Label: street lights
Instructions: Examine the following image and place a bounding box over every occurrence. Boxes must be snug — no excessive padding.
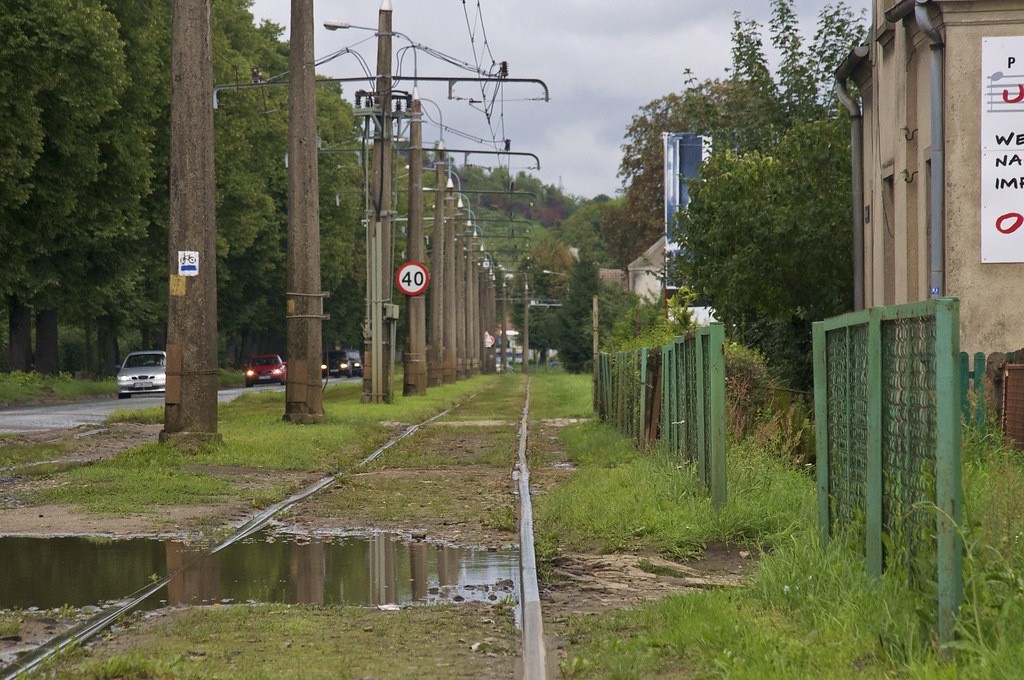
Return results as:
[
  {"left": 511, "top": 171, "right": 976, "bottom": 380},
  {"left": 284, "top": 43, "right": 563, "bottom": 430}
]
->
[
  {"left": 543, "top": 270, "right": 569, "bottom": 297},
  {"left": 322, "top": 17, "right": 392, "bottom": 403}
]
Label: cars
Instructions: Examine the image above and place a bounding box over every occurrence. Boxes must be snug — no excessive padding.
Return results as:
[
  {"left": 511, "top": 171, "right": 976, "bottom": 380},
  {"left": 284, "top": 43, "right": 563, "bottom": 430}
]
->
[
  {"left": 321, "top": 349, "right": 352, "bottom": 379},
  {"left": 244, "top": 354, "right": 287, "bottom": 388},
  {"left": 113, "top": 351, "right": 167, "bottom": 399},
  {"left": 344, "top": 348, "right": 364, "bottom": 377}
]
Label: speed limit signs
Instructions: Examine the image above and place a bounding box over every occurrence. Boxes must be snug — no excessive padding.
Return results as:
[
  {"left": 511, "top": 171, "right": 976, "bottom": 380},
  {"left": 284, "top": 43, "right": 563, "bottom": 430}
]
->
[{"left": 394, "top": 261, "right": 431, "bottom": 296}]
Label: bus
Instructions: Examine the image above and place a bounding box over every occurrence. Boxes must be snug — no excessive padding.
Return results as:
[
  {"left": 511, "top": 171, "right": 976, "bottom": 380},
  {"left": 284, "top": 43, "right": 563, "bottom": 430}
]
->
[{"left": 497, "top": 330, "right": 559, "bottom": 365}]
